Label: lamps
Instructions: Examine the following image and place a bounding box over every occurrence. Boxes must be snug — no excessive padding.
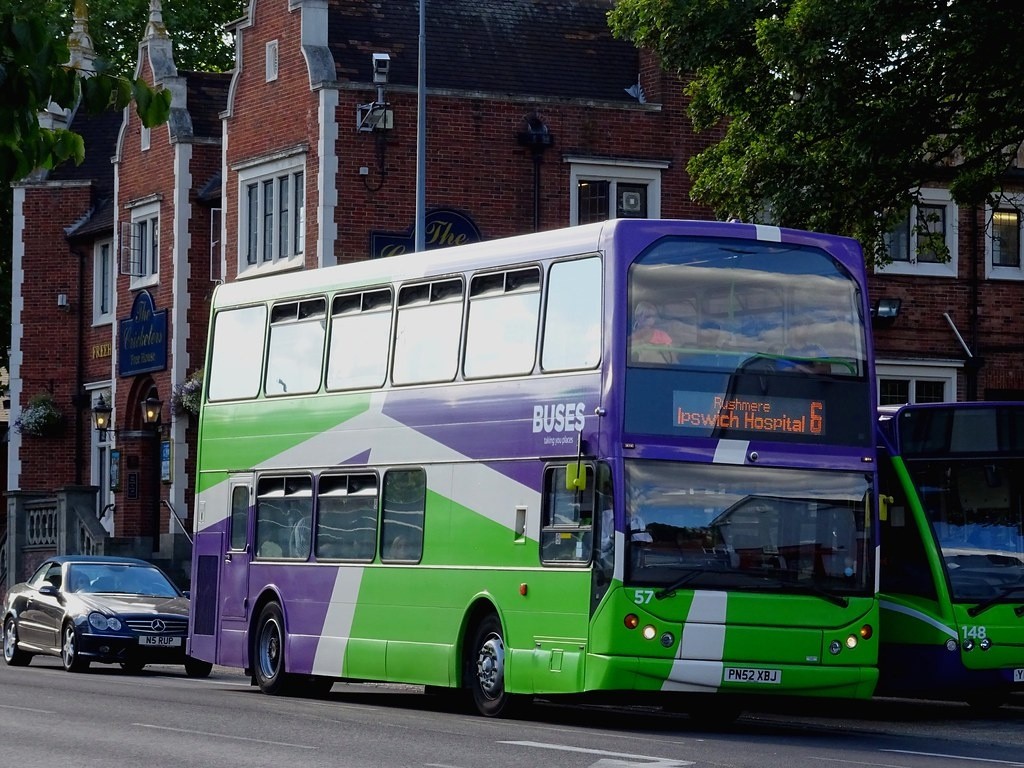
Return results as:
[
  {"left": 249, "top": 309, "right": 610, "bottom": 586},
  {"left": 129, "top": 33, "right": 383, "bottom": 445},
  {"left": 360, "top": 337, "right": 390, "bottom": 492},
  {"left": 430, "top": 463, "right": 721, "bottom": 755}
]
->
[
  {"left": 869, "top": 297, "right": 903, "bottom": 327},
  {"left": 89, "top": 393, "right": 118, "bottom": 436},
  {"left": 57, "top": 293, "right": 68, "bottom": 310},
  {"left": 139, "top": 389, "right": 172, "bottom": 428}
]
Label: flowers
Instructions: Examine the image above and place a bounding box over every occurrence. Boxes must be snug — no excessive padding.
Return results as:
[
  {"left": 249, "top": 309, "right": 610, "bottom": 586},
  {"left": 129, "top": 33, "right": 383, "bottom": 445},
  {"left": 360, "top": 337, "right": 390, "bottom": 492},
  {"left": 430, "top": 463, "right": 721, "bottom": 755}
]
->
[
  {"left": 169, "top": 365, "right": 205, "bottom": 416},
  {"left": 8, "top": 392, "right": 64, "bottom": 436}
]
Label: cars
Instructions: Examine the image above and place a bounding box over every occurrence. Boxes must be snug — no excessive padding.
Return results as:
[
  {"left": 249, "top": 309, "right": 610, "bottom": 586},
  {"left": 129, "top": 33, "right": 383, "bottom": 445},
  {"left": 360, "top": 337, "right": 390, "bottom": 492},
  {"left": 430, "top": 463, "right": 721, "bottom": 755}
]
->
[{"left": 2, "top": 554, "right": 212, "bottom": 678}]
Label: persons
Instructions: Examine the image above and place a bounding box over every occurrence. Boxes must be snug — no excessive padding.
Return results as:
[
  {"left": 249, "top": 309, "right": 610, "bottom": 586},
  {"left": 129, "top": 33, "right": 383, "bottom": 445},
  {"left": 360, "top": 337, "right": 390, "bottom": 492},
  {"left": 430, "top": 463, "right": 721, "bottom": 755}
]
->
[
  {"left": 630, "top": 301, "right": 831, "bottom": 372},
  {"left": 600, "top": 487, "right": 646, "bottom": 567}
]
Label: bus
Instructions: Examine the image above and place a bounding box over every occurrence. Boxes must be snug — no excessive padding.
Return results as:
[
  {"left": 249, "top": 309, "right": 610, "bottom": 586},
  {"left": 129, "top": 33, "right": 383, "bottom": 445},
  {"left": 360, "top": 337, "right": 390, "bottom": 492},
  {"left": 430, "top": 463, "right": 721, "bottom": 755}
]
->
[
  {"left": 876, "top": 401, "right": 1024, "bottom": 711},
  {"left": 186, "top": 218, "right": 884, "bottom": 721}
]
